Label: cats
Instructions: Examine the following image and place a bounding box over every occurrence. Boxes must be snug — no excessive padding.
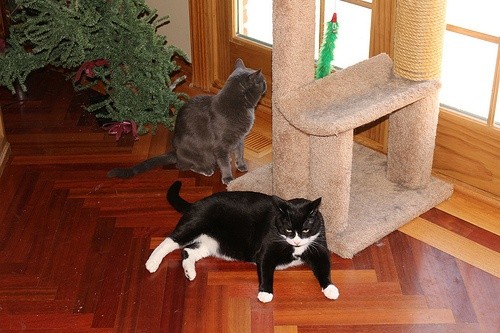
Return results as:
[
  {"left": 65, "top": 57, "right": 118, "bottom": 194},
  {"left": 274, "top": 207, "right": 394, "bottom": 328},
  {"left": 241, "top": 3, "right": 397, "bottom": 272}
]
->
[
  {"left": 144, "top": 179, "right": 340, "bottom": 304},
  {"left": 105, "top": 56, "right": 271, "bottom": 186}
]
